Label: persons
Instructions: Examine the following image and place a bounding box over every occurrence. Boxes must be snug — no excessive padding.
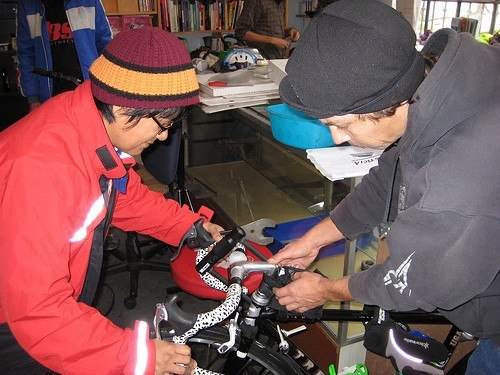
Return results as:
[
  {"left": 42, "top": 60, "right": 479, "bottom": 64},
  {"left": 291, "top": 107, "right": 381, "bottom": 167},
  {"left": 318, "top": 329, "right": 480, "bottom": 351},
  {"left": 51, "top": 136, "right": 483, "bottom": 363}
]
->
[
  {"left": 266, "top": 0, "right": 500, "bottom": 375},
  {"left": 235, "top": 0, "right": 301, "bottom": 59},
  {"left": 0, "top": 24, "right": 225, "bottom": 375},
  {"left": 16, "top": 0, "right": 114, "bottom": 113}
]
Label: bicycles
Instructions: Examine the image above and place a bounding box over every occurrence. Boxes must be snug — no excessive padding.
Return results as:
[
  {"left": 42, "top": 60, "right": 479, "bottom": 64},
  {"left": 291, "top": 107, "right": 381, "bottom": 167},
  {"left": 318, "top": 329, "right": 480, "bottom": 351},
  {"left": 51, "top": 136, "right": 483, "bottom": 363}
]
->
[{"left": 150, "top": 217, "right": 459, "bottom": 375}]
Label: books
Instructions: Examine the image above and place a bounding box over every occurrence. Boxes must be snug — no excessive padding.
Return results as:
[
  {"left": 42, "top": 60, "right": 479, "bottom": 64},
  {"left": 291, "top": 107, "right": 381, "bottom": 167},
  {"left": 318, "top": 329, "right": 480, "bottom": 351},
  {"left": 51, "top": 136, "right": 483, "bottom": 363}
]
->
[
  {"left": 451, "top": 17, "right": 478, "bottom": 36},
  {"left": 138, "top": 0, "right": 245, "bottom": 33}
]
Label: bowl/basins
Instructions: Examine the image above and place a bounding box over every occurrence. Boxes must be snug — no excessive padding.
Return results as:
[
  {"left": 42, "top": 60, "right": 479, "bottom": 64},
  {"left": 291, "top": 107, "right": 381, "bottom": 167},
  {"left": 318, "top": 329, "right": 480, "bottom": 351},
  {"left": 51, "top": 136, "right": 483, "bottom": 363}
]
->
[{"left": 264, "top": 104, "right": 336, "bottom": 149}]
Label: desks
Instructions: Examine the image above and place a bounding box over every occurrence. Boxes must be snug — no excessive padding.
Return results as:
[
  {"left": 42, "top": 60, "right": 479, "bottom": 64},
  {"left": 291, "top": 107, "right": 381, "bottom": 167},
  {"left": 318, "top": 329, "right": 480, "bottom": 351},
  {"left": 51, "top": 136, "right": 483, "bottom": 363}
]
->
[{"left": 232, "top": 107, "right": 409, "bottom": 347}]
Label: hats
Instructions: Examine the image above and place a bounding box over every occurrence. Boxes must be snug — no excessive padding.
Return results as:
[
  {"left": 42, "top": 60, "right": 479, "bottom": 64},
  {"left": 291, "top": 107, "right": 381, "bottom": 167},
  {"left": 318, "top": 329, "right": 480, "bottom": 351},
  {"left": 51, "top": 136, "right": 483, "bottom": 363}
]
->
[
  {"left": 279, "top": 0, "right": 425, "bottom": 118},
  {"left": 88, "top": 26, "right": 200, "bottom": 109}
]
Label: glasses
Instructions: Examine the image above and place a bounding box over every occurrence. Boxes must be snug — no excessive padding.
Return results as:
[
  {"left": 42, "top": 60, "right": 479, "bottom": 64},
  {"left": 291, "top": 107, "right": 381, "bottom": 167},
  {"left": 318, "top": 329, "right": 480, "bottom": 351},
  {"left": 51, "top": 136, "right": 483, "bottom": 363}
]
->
[{"left": 148, "top": 113, "right": 174, "bottom": 135}]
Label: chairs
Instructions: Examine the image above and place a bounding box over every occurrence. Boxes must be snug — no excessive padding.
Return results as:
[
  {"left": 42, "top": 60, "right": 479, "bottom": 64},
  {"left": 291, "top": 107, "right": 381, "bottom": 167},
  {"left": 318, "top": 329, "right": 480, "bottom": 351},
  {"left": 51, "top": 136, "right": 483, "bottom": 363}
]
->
[{"left": 95, "top": 112, "right": 186, "bottom": 308}]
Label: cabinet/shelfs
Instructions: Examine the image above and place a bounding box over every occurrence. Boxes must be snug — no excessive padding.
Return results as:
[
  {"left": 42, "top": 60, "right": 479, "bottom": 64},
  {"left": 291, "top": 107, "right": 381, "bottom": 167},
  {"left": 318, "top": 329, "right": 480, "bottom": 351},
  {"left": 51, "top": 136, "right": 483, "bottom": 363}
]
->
[{"left": 101, "top": 0, "right": 289, "bottom": 60}]
